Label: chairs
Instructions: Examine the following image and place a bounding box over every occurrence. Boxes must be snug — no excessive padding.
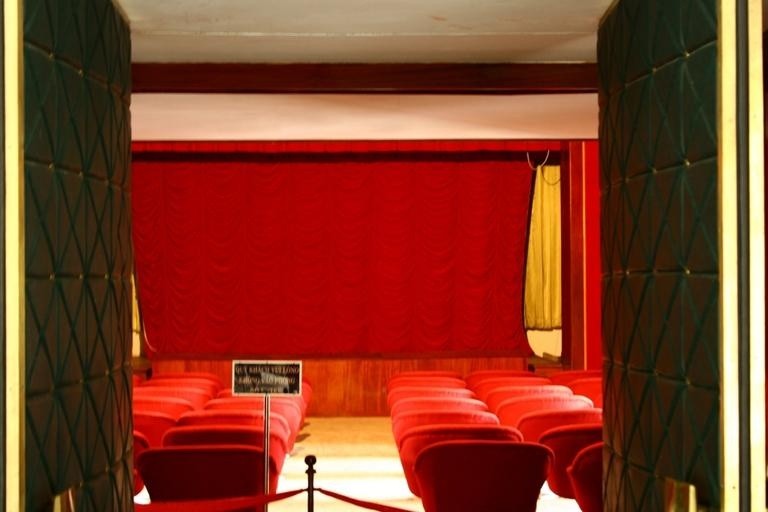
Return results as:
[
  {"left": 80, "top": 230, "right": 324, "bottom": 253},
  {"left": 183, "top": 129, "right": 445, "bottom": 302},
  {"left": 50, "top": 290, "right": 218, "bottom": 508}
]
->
[
  {"left": 126, "top": 365, "right": 316, "bottom": 509},
  {"left": 380, "top": 365, "right": 607, "bottom": 512}
]
